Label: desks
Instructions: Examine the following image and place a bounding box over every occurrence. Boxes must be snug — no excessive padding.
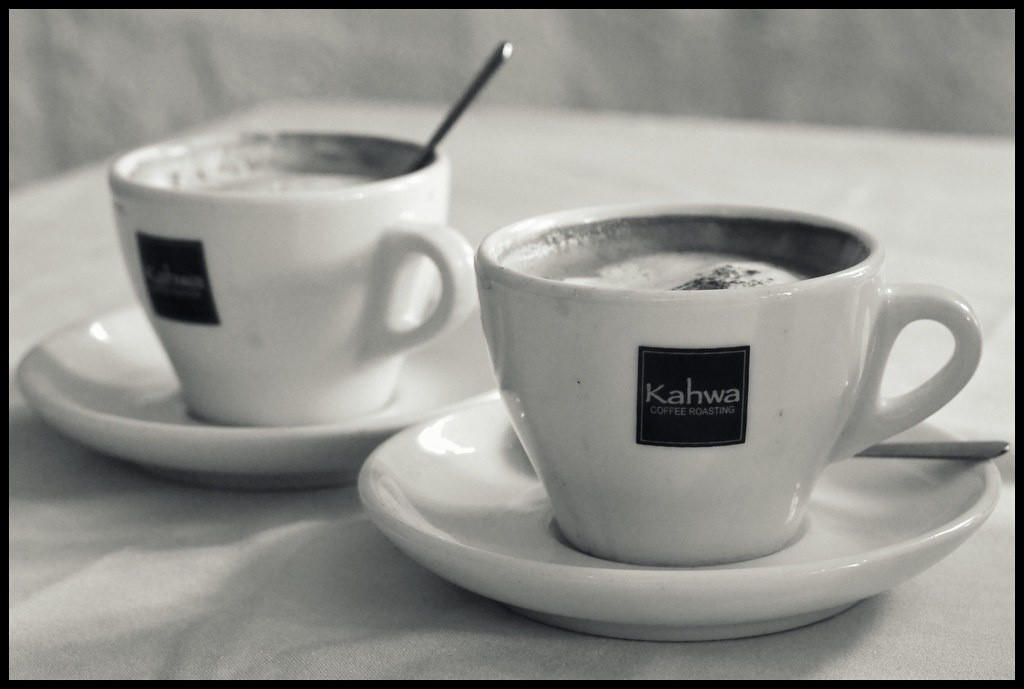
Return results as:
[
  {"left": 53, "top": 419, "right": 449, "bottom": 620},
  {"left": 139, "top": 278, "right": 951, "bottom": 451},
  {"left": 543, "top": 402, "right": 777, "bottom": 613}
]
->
[{"left": 9, "top": 99, "right": 1016, "bottom": 681}]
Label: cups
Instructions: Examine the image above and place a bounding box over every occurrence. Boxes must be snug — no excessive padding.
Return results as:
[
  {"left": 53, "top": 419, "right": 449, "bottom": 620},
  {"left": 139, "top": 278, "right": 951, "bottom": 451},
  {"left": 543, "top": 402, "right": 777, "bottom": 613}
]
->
[
  {"left": 476, "top": 206, "right": 983, "bottom": 565},
  {"left": 107, "top": 129, "right": 476, "bottom": 426}
]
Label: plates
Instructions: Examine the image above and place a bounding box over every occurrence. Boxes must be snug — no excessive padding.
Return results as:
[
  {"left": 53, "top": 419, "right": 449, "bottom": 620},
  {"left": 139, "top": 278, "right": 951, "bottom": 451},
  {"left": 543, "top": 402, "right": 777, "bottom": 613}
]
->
[
  {"left": 13, "top": 305, "right": 503, "bottom": 485},
  {"left": 358, "top": 394, "right": 1001, "bottom": 644}
]
